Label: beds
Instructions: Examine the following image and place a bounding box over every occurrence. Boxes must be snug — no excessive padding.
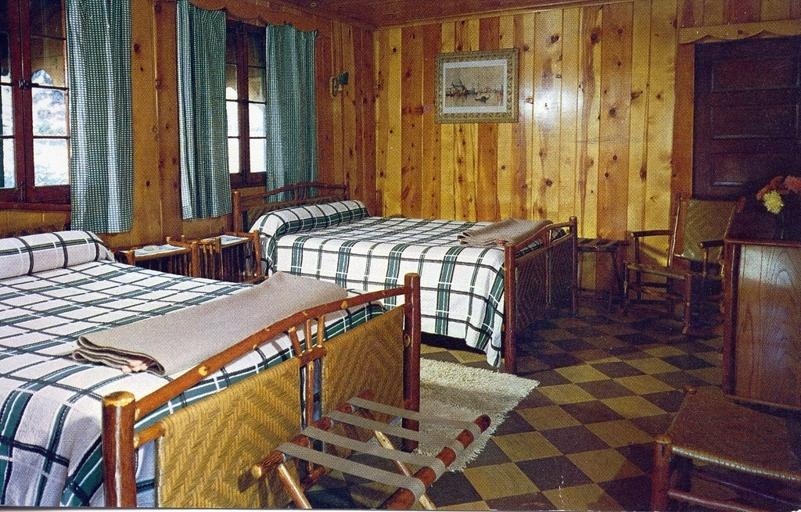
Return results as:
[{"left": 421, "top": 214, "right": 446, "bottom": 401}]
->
[
  {"left": 230, "top": 181, "right": 580, "bottom": 374},
  {"left": 0, "top": 208, "right": 421, "bottom": 507}
]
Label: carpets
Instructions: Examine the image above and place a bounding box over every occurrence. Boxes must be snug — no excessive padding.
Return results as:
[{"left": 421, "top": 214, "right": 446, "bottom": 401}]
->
[{"left": 365, "top": 351, "right": 540, "bottom": 473}]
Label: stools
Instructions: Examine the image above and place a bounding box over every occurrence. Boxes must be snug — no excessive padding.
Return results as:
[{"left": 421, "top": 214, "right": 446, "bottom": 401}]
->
[{"left": 248, "top": 389, "right": 490, "bottom": 511}]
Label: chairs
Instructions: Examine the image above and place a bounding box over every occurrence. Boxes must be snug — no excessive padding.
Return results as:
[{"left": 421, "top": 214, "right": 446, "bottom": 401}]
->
[{"left": 618, "top": 194, "right": 743, "bottom": 345}]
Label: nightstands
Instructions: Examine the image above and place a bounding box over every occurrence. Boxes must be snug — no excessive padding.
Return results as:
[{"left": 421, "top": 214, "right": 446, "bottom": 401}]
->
[{"left": 116, "top": 228, "right": 263, "bottom": 284}]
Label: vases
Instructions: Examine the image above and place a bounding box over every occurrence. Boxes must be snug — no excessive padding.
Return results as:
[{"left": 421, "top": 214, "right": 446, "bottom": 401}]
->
[{"left": 771, "top": 210, "right": 789, "bottom": 239}]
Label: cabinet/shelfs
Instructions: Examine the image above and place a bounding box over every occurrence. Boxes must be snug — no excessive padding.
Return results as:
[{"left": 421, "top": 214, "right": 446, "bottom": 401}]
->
[
  {"left": 691, "top": 35, "right": 801, "bottom": 202},
  {"left": 718, "top": 243, "right": 801, "bottom": 412}
]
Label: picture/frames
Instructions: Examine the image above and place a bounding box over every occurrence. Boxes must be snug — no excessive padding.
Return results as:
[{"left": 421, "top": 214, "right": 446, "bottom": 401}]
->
[{"left": 433, "top": 48, "right": 520, "bottom": 124}]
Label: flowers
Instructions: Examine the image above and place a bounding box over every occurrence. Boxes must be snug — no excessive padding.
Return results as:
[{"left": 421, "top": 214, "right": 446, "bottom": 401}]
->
[{"left": 753, "top": 174, "right": 801, "bottom": 216}]
